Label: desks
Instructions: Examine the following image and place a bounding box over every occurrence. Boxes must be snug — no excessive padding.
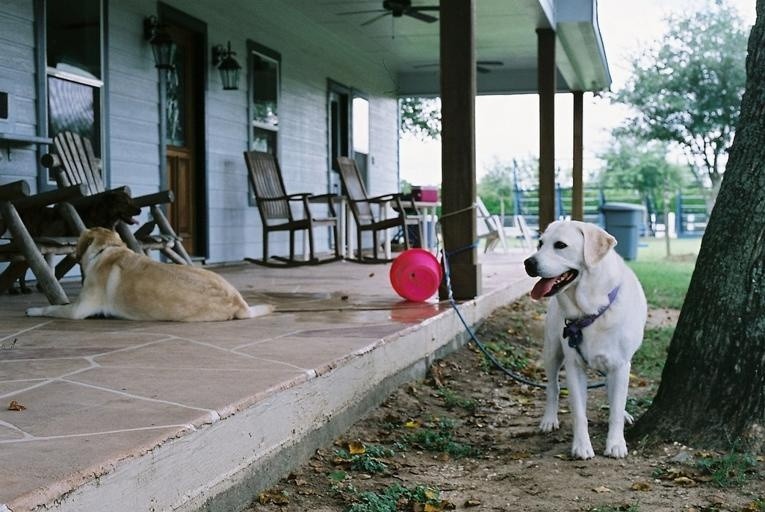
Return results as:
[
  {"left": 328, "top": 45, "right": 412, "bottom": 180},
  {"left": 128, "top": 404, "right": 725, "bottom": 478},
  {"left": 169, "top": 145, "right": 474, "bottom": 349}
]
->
[{"left": 390, "top": 200, "right": 442, "bottom": 253}]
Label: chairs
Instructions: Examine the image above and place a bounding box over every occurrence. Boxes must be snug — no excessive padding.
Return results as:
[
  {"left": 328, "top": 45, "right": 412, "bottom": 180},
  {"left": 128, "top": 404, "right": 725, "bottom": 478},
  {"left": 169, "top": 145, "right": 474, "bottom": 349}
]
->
[
  {"left": 243, "top": 150, "right": 341, "bottom": 268},
  {"left": 0, "top": 91, "right": 91, "bottom": 309},
  {"left": 337, "top": 156, "right": 422, "bottom": 265},
  {"left": 39, "top": 130, "right": 194, "bottom": 266}
]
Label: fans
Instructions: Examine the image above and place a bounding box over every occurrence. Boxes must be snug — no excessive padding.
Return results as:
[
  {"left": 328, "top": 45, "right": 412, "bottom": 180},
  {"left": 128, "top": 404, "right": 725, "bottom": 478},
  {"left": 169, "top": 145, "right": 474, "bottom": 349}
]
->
[
  {"left": 413, "top": 60, "right": 503, "bottom": 73},
  {"left": 331, "top": 0, "right": 440, "bottom": 27}
]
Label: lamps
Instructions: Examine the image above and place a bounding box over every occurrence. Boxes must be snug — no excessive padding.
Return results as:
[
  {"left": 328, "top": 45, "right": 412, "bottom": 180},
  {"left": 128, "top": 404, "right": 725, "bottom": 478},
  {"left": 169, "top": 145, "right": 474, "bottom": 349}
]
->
[
  {"left": 212, "top": 40, "right": 241, "bottom": 90},
  {"left": 144, "top": 14, "right": 181, "bottom": 70}
]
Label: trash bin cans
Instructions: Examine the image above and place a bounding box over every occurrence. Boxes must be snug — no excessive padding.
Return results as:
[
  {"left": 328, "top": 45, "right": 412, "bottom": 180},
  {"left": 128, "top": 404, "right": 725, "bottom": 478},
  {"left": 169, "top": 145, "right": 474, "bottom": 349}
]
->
[
  {"left": 596, "top": 202, "right": 646, "bottom": 260},
  {"left": 405, "top": 214, "right": 438, "bottom": 248}
]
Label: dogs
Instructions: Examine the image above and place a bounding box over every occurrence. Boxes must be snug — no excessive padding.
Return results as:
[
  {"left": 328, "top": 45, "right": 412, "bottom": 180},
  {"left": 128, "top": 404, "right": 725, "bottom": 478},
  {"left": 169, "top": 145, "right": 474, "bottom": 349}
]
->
[
  {"left": 25, "top": 227, "right": 279, "bottom": 323},
  {"left": 523, "top": 220, "right": 649, "bottom": 461}
]
